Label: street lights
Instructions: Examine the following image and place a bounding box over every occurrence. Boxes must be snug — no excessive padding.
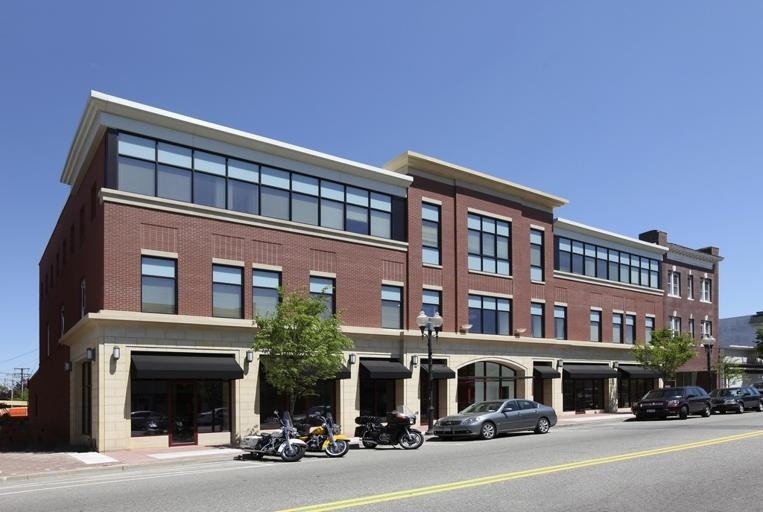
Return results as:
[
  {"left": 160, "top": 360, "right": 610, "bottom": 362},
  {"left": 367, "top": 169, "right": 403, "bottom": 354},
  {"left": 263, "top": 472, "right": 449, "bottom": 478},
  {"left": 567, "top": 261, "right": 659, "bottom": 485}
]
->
[
  {"left": 416, "top": 309, "right": 444, "bottom": 436},
  {"left": 702, "top": 335, "right": 716, "bottom": 392}
]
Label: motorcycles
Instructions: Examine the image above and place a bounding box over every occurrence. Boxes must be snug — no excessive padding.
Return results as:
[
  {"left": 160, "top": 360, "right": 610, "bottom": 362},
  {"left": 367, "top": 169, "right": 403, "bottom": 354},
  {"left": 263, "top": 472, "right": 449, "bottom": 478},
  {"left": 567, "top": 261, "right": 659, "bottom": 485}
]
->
[{"left": 234, "top": 405, "right": 424, "bottom": 462}]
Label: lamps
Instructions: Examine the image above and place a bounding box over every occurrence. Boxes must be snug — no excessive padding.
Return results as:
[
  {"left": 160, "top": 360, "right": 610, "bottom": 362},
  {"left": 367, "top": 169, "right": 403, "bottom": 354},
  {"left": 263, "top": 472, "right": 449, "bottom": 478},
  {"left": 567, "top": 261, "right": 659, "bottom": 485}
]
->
[
  {"left": 246, "top": 350, "right": 253, "bottom": 363},
  {"left": 411, "top": 355, "right": 417, "bottom": 365},
  {"left": 348, "top": 353, "right": 355, "bottom": 364},
  {"left": 112, "top": 346, "right": 120, "bottom": 360},
  {"left": 614, "top": 361, "right": 619, "bottom": 368},
  {"left": 558, "top": 359, "right": 563, "bottom": 367}
]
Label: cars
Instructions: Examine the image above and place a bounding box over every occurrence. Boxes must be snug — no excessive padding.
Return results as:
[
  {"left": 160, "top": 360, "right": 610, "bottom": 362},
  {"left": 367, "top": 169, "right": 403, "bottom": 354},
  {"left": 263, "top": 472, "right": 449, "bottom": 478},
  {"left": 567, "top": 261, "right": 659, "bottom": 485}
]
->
[
  {"left": 198, "top": 408, "right": 229, "bottom": 426},
  {"left": 433, "top": 399, "right": 557, "bottom": 440},
  {"left": 631, "top": 381, "right": 763, "bottom": 420},
  {"left": 131, "top": 410, "right": 167, "bottom": 431}
]
